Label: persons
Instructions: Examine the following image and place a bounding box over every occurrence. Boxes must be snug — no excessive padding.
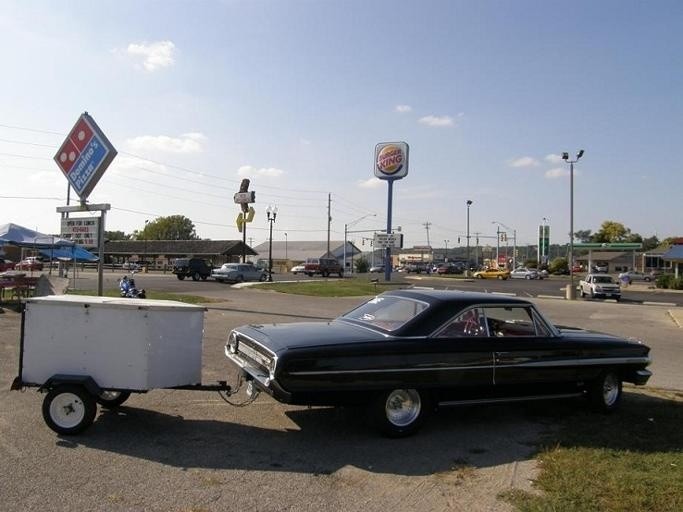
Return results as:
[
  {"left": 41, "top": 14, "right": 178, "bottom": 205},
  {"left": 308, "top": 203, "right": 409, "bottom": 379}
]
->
[
  {"left": 0, "top": 258, "right": 5, "bottom": 264},
  {"left": 433, "top": 265, "right": 437, "bottom": 273},
  {"left": 425, "top": 264, "right": 432, "bottom": 274},
  {"left": 119, "top": 275, "right": 128, "bottom": 297}
]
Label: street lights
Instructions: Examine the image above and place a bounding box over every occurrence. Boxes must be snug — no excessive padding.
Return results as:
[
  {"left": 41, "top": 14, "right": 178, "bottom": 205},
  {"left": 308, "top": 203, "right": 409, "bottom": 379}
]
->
[
  {"left": 562, "top": 149, "right": 584, "bottom": 284},
  {"left": 266, "top": 207, "right": 278, "bottom": 282},
  {"left": 466, "top": 200, "right": 472, "bottom": 270}
]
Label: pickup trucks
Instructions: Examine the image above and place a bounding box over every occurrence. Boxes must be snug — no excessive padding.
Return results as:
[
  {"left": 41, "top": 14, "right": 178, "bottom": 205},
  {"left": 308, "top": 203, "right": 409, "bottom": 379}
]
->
[{"left": 579, "top": 273, "right": 621, "bottom": 302}]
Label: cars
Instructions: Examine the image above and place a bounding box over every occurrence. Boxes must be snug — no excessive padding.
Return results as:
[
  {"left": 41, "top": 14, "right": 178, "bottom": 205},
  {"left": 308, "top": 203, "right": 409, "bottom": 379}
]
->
[
  {"left": 618, "top": 271, "right": 662, "bottom": 283},
  {"left": 594, "top": 263, "right": 608, "bottom": 273},
  {"left": 472, "top": 266, "right": 548, "bottom": 280},
  {"left": 290, "top": 258, "right": 344, "bottom": 278},
  {"left": 224, "top": 289, "right": 652, "bottom": 438},
  {"left": 392, "top": 262, "right": 464, "bottom": 274},
  {"left": 0, "top": 256, "right": 42, "bottom": 272},
  {"left": 370, "top": 264, "right": 386, "bottom": 273},
  {"left": 172, "top": 257, "right": 268, "bottom": 283}
]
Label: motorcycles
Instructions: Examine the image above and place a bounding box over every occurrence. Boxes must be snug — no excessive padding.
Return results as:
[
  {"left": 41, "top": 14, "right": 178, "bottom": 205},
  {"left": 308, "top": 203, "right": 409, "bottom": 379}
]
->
[{"left": 117, "top": 275, "right": 146, "bottom": 298}]
